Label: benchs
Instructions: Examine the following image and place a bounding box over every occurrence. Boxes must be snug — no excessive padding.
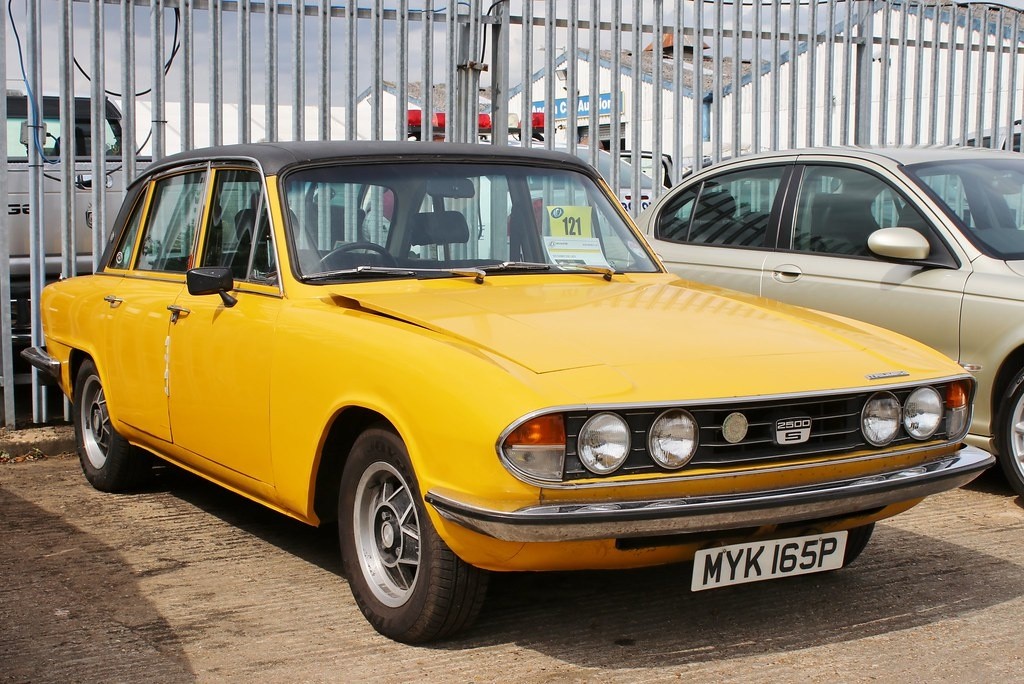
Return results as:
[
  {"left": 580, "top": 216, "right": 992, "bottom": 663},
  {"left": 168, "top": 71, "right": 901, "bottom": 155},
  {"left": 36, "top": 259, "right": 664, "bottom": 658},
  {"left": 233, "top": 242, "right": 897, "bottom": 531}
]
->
[
  {"left": 410, "top": 211, "right": 503, "bottom": 269},
  {"left": 694, "top": 192, "right": 770, "bottom": 246},
  {"left": 152, "top": 249, "right": 322, "bottom": 278}
]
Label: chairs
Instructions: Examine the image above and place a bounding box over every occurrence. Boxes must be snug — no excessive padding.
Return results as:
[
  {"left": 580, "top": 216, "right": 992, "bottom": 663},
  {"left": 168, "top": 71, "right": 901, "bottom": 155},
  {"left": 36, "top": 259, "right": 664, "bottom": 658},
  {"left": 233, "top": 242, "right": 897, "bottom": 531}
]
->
[
  {"left": 814, "top": 202, "right": 869, "bottom": 256},
  {"left": 897, "top": 203, "right": 929, "bottom": 233},
  {"left": 234, "top": 208, "right": 300, "bottom": 273},
  {"left": 55, "top": 127, "right": 86, "bottom": 156}
]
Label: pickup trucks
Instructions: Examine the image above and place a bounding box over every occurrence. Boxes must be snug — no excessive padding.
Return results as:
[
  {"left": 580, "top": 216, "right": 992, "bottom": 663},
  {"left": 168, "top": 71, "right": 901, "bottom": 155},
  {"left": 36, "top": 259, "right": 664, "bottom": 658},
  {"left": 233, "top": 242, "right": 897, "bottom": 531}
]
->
[{"left": 6, "top": 91, "right": 248, "bottom": 346}]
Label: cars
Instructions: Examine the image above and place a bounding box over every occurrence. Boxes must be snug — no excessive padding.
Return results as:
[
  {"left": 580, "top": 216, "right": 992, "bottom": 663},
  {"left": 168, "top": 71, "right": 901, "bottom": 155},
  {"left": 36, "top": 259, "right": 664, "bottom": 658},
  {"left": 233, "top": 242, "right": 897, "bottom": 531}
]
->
[
  {"left": 303, "top": 106, "right": 768, "bottom": 268},
  {"left": 829, "top": 118, "right": 1024, "bottom": 233},
  {"left": 602, "top": 146, "right": 1024, "bottom": 507},
  {"left": 15, "top": 140, "right": 999, "bottom": 647}
]
